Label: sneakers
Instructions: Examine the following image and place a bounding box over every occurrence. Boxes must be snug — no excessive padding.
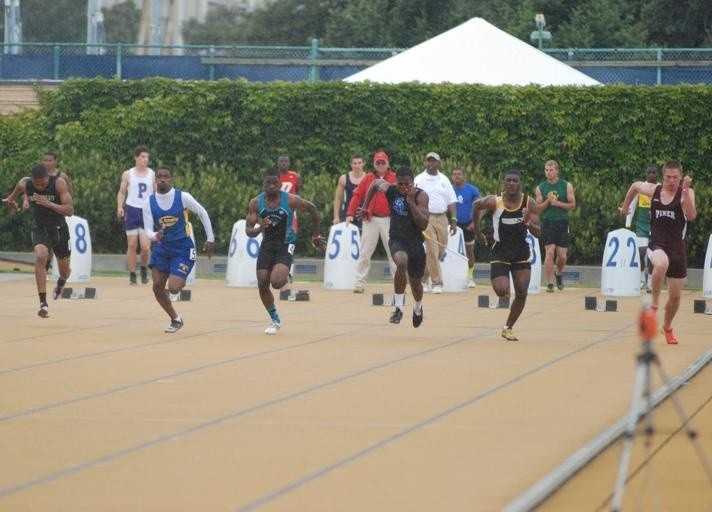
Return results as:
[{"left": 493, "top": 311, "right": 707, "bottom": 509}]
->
[
  {"left": 660, "top": 325, "right": 679, "bottom": 344},
  {"left": 38, "top": 277, "right": 184, "bottom": 332},
  {"left": 264, "top": 319, "right": 282, "bottom": 334},
  {"left": 353, "top": 270, "right": 563, "bottom": 341}
]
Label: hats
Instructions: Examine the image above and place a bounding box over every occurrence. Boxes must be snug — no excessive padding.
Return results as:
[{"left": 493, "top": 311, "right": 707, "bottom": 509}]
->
[
  {"left": 425, "top": 152, "right": 441, "bottom": 162},
  {"left": 373, "top": 152, "right": 389, "bottom": 161}
]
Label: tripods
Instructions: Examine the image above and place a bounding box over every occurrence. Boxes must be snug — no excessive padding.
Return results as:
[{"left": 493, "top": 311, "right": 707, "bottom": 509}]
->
[{"left": 610, "top": 300, "right": 712, "bottom": 512}]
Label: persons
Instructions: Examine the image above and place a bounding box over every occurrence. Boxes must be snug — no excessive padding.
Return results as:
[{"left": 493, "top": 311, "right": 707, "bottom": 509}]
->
[
  {"left": 620, "top": 159, "right": 698, "bottom": 344},
  {"left": 472, "top": 173, "right": 542, "bottom": 342},
  {"left": 625, "top": 166, "right": 661, "bottom": 294},
  {"left": 332, "top": 153, "right": 486, "bottom": 330},
  {"left": 22, "top": 151, "right": 72, "bottom": 281},
  {"left": 117, "top": 145, "right": 157, "bottom": 286},
  {"left": 143, "top": 166, "right": 215, "bottom": 333},
  {"left": 275, "top": 154, "right": 304, "bottom": 284},
  {"left": 245, "top": 167, "right": 327, "bottom": 334},
  {"left": 535, "top": 159, "right": 576, "bottom": 293},
  {"left": 2, "top": 163, "right": 75, "bottom": 318}
]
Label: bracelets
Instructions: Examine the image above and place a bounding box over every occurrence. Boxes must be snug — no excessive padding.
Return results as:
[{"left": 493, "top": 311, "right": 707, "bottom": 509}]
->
[
  {"left": 683, "top": 188, "right": 690, "bottom": 193},
  {"left": 556, "top": 201, "right": 560, "bottom": 208},
  {"left": 312, "top": 234, "right": 320, "bottom": 241},
  {"left": 525, "top": 219, "right": 532, "bottom": 226}
]
